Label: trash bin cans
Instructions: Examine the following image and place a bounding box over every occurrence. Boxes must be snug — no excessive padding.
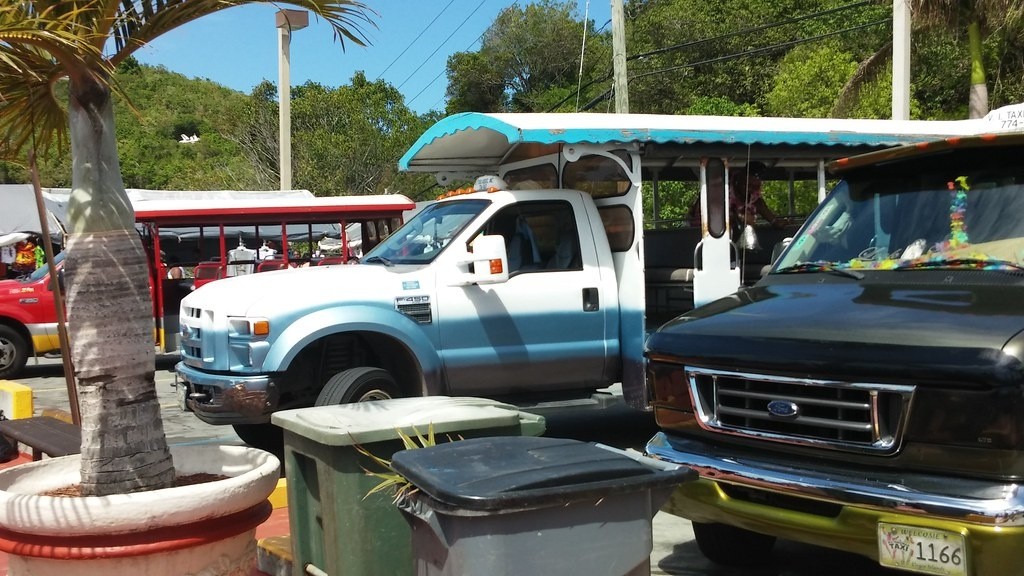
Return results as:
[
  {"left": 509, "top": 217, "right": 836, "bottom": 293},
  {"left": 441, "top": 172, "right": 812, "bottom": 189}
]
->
[
  {"left": 390, "top": 438, "right": 701, "bottom": 576},
  {"left": 271, "top": 395, "right": 547, "bottom": 576}
]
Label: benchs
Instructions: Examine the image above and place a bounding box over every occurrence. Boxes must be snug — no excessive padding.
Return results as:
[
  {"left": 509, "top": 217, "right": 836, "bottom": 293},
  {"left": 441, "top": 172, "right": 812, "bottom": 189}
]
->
[
  {"left": 163, "top": 257, "right": 343, "bottom": 316},
  {"left": 0, "top": 416, "right": 82, "bottom": 461},
  {"left": 645, "top": 269, "right": 695, "bottom": 311},
  {"left": 741, "top": 225, "right": 800, "bottom": 281}
]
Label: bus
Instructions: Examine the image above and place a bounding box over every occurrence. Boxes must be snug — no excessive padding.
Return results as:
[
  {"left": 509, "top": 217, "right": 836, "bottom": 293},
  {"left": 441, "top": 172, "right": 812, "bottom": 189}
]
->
[
  {"left": 0, "top": 195, "right": 414, "bottom": 381},
  {"left": 174, "top": 110, "right": 993, "bottom": 462}
]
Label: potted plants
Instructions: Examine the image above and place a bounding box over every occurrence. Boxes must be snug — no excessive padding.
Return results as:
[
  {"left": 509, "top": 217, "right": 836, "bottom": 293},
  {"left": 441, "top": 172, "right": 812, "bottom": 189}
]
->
[{"left": 1, "top": 0, "right": 380, "bottom": 575}]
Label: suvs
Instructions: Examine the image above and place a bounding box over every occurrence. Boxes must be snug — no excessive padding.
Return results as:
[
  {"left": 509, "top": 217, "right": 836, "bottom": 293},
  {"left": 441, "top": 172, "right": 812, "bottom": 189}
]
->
[{"left": 645, "top": 129, "right": 1024, "bottom": 576}]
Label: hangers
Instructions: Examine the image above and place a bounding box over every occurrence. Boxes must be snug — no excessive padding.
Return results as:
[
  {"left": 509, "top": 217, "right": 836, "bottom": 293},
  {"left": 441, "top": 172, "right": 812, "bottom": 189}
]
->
[
  {"left": 254, "top": 241, "right": 274, "bottom": 251},
  {"left": 234, "top": 242, "right": 248, "bottom": 252}
]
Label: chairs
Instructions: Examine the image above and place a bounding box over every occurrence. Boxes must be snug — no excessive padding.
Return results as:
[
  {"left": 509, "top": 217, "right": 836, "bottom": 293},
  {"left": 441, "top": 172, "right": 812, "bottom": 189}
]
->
[
  {"left": 507, "top": 233, "right": 529, "bottom": 272},
  {"left": 545, "top": 231, "right": 578, "bottom": 270}
]
